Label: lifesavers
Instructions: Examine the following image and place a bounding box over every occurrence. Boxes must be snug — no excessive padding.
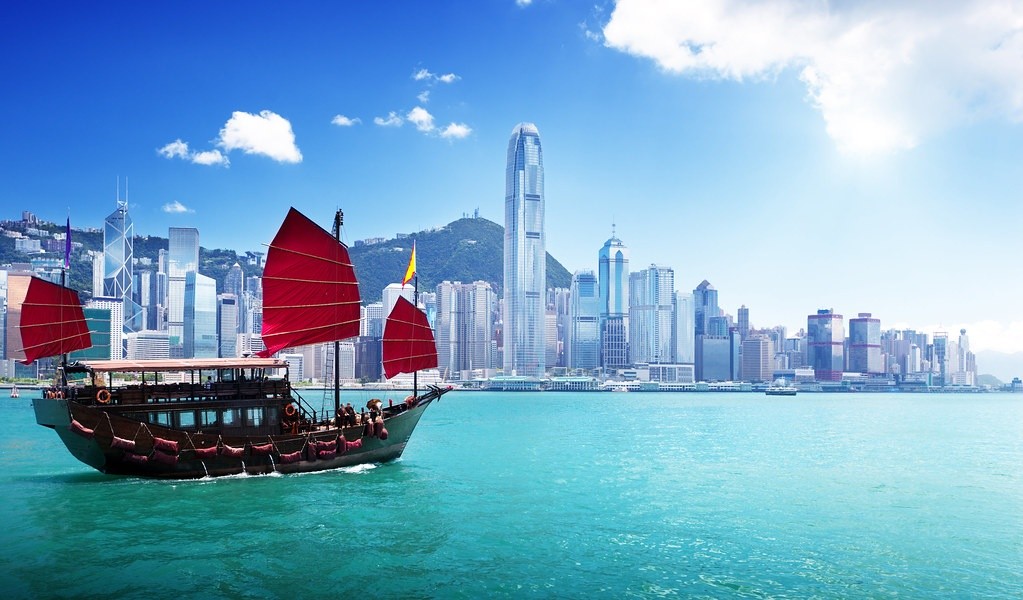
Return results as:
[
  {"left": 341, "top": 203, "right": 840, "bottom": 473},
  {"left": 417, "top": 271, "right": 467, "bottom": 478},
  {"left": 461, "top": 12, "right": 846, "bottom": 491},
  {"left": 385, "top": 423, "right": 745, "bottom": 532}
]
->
[
  {"left": 46, "top": 391, "right": 55, "bottom": 398},
  {"left": 97, "top": 390, "right": 110, "bottom": 403},
  {"left": 285, "top": 403, "right": 296, "bottom": 416},
  {"left": 57, "top": 391, "right": 65, "bottom": 400}
]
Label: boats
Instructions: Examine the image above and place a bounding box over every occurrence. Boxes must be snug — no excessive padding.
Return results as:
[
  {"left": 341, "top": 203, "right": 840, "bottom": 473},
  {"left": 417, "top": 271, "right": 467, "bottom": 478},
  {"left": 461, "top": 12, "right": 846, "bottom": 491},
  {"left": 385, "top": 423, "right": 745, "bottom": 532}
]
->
[
  {"left": 10, "top": 384, "right": 19, "bottom": 398},
  {"left": 8, "top": 207, "right": 453, "bottom": 479}
]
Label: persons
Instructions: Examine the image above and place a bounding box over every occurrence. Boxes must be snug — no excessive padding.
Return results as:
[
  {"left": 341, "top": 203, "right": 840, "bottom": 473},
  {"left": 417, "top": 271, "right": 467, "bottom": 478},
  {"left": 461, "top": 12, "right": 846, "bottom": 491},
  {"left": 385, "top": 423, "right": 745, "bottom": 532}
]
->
[
  {"left": 207, "top": 376, "right": 214, "bottom": 383},
  {"left": 345, "top": 403, "right": 354, "bottom": 428},
  {"left": 337, "top": 403, "right": 347, "bottom": 429},
  {"left": 241, "top": 371, "right": 246, "bottom": 380},
  {"left": 47, "top": 388, "right": 65, "bottom": 399}
]
